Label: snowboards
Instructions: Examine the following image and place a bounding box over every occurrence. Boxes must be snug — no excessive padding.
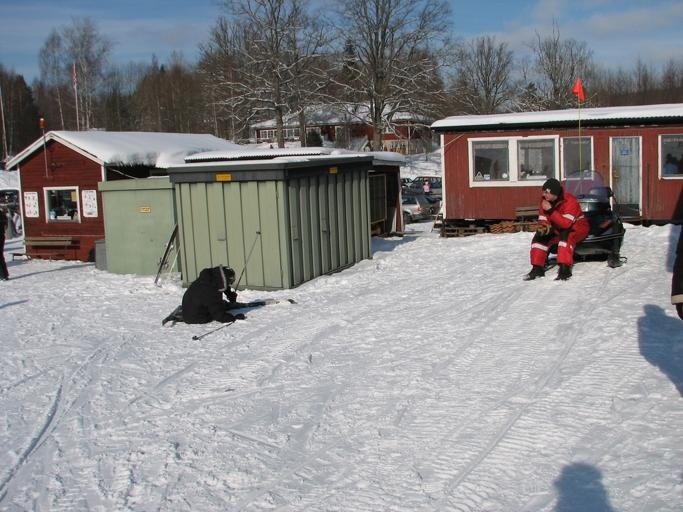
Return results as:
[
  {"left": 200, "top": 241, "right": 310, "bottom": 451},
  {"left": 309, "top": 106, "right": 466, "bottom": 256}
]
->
[{"left": 154, "top": 225, "right": 178, "bottom": 283}]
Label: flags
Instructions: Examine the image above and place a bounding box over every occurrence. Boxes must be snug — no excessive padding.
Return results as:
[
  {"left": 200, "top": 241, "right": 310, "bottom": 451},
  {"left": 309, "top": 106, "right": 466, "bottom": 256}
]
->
[{"left": 572, "top": 80, "right": 589, "bottom": 102}]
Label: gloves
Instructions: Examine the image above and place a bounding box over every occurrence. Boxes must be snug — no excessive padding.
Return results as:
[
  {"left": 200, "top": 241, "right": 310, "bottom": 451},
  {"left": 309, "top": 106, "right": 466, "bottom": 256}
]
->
[
  {"left": 536, "top": 223, "right": 552, "bottom": 238},
  {"left": 219, "top": 312, "right": 236, "bottom": 324},
  {"left": 226, "top": 291, "right": 237, "bottom": 302}
]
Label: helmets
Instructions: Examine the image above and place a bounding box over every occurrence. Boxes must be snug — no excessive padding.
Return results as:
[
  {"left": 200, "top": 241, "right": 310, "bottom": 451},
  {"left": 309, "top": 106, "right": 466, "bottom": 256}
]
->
[{"left": 222, "top": 265, "right": 236, "bottom": 279}]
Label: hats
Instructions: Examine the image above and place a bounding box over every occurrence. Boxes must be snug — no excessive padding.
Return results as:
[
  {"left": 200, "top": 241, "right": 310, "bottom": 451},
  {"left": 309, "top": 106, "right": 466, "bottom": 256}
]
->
[{"left": 541, "top": 177, "right": 561, "bottom": 195}]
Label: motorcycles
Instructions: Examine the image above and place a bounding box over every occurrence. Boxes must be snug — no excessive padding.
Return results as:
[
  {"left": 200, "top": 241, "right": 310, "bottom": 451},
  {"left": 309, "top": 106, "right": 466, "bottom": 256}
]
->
[{"left": 549, "top": 167, "right": 626, "bottom": 263}]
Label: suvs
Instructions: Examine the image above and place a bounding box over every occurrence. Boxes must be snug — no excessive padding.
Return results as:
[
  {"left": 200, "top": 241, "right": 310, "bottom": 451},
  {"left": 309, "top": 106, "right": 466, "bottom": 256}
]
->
[{"left": 402, "top": 176, "right": 441, "bottom": 203}]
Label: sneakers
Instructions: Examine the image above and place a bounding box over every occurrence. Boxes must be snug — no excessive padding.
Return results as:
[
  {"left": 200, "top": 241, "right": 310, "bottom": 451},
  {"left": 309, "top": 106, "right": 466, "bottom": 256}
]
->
[
  {"left": 553, "top": 265, "right": 574, "bottom": 282},
  {"left": 522, "top": 265, "right": 546, "bottom": 282}
]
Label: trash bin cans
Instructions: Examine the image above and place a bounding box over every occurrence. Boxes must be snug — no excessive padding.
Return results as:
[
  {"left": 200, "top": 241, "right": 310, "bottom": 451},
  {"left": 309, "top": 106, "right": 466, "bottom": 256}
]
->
[{"left": 94, "top": 240, "right": 106, "bottom": 270}]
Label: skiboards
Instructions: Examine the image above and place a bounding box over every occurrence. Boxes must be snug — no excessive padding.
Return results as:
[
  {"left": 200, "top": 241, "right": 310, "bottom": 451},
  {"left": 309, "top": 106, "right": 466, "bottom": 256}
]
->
[{"left": 162, "top": 299, "right": 298, "bottom": 326}]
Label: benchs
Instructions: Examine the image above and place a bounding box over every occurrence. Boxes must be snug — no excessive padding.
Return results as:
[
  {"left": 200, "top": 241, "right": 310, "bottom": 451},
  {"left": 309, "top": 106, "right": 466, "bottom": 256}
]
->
[
  {"left": 7, "top": 237, "right": 73, "bottom": 262},
  {"left": 515, "top": 203, "right": 540, "bottom": 232}
]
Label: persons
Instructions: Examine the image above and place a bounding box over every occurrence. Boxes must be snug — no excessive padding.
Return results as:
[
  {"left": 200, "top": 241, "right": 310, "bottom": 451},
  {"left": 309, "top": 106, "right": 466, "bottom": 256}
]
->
[
  {"left": 523, "top": 182, "right": 589, "bottom": 281},
  {"left": 422, "top": 181, "right": 430, "bottom": 194},
  {"left": 671, "top": 227, "right": 683, "bottom": 322},
  {"left": 0, "top": 211, "right": 13, "bottom": 282},
  {"left": 182, "top": 265, "right": 256, "bottom": 324}
]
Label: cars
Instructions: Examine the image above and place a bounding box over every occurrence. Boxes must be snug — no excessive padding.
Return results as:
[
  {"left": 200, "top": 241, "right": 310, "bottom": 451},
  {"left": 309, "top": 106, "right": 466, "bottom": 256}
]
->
[
  {"left": 0, "top": 189, "right": 19, "bottom": 211},
  {"left": 400, "top": 178, "right": 412, "bottom": 185}
]
